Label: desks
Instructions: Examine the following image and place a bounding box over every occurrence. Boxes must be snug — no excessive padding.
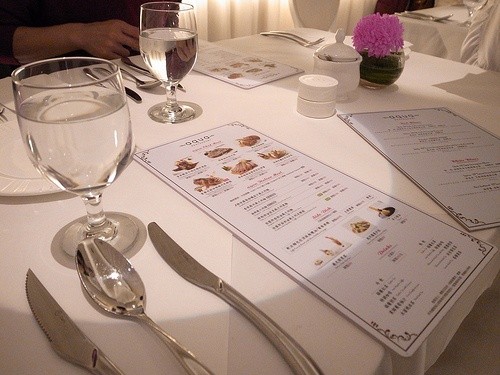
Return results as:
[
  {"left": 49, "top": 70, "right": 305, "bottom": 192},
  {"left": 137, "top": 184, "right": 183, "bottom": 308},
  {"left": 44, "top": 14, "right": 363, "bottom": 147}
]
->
[
  {"left": 391, "top": 6, "right": 477, "bottom": 63},
  {"left": 0, "top": 27, "right": 500, "bottom": 375}
]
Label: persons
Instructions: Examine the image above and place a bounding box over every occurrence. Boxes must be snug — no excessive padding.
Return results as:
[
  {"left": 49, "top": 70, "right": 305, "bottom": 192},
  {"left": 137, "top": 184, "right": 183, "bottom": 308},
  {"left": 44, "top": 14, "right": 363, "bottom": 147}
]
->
[{"left": 0, "top": 0, "right": 197, "bottom": 82}]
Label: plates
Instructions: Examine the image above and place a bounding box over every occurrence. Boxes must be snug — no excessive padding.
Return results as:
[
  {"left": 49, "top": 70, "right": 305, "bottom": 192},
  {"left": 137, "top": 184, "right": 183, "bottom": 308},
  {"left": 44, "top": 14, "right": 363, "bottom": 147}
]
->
[{"left": 0, "top": 74, "right": 69, "bottom": 196}]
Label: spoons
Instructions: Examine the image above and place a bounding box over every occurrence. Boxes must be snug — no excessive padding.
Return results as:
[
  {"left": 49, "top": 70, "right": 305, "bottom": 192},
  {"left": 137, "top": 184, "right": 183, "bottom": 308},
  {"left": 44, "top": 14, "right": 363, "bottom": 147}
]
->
[
  {"left": 75, "top": 239, "right": 215, "bottom": 375},
  {"left": 401, "top": 12, "right": 452, "bottom": 21},
  {"left": 120, "top": 67, "right": 162, "bottom": 89}
]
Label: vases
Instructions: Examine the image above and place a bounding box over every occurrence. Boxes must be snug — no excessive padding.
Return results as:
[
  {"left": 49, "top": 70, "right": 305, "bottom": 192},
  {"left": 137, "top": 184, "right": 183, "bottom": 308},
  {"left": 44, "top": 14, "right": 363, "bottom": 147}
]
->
[{"left": 358, "top": 47, "right": 405, "bottom": 87}]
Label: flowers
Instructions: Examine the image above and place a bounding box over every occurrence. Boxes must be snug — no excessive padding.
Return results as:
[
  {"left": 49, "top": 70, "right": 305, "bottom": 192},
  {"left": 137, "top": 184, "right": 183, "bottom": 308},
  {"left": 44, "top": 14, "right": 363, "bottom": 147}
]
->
[{"left": 351, "top": 12, "right": 405, "bottom": 58}]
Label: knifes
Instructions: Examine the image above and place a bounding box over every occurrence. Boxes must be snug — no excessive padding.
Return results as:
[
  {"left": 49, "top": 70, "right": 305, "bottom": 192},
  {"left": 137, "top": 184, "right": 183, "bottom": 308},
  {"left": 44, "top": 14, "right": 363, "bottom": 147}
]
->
[
  {"left": 121, "top": 55, "right": 183, "bottom": 89},
  {"left": 25, "top": 268, "right": 126, "bottom": 375},
  {"left": 148, "top": 221, "right": 324, "bottom": 375},
  {"left": 92, "top": 67, "right": 142, "bottom": 102}
]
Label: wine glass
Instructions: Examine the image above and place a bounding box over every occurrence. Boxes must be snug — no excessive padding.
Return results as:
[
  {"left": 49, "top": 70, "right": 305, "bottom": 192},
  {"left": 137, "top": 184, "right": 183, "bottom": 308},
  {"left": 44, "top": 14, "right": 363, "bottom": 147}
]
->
[
  {"left": 139, "top": 2, "right": 198, "bottom": 124},
  {"left": 11, "top": 56, "right": 138, "bottom": 255},
  {"left": 462, "top": 0, "right": 487, "bottom": 26}
]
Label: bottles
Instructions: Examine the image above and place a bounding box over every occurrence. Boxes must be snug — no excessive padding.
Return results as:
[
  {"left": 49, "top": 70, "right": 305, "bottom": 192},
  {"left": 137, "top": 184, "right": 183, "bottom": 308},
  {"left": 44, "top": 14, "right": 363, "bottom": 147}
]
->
[{"left": 312, "top": 29, "right": 362, "bottom": 102}]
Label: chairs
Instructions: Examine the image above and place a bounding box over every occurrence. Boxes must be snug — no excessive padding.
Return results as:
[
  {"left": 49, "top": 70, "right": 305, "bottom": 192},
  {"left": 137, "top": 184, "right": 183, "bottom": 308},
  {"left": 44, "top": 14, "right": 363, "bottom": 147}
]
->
[{"left": 287, "top": 0, "right": 340, "bottom": 31}]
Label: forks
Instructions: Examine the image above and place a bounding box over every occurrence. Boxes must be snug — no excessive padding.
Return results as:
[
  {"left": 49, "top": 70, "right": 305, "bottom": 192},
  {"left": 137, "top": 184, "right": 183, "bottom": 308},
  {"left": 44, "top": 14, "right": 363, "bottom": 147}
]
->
[{"left": 260, "top": 30, "right": 325, "bottom": 47}]
